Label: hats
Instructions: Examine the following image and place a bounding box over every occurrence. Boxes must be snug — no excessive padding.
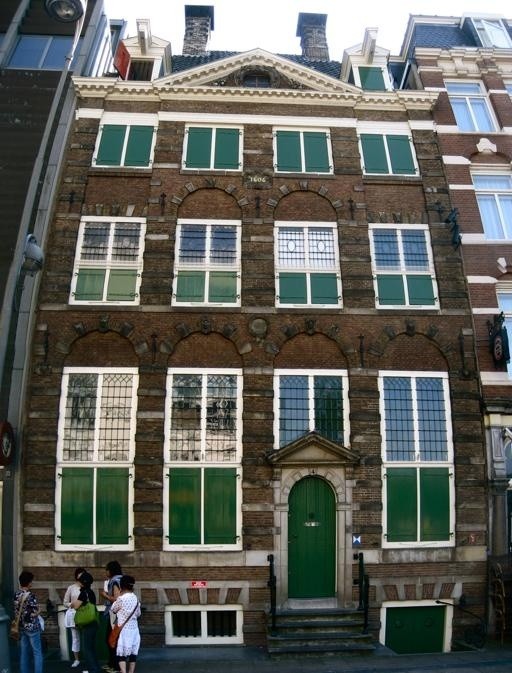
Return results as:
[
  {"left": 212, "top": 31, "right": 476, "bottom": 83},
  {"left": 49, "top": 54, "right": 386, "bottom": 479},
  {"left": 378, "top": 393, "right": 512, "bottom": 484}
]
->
[{"left": 77, "top": 573, "right": 93, "bottom": 585}]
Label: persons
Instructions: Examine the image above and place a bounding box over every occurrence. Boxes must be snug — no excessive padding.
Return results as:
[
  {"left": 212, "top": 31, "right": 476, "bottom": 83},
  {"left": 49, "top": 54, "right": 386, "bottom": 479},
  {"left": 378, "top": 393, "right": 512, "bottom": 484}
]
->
[
  {"left": 70, "top": 572, "right": 103, "bottom": 673},
  {"left": 62, "top": 568, "right": 87, "bottom": 669},
  {"left": 100, "top": 560, "right": 124, "bottom": 673},
  {"left": 110, "top": 574, "right": 142, "bottom": 673},
  {"left": 12, "top": 571, "right": 44, "bottom": 673}
]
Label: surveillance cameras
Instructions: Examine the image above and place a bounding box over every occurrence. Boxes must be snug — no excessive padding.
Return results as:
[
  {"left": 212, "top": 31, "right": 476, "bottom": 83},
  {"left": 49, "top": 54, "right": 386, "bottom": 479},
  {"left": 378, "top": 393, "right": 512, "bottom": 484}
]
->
[
  {"left": 444, "top": 206, "right": 460, "bottom": 223},
  {"left": 20, "top": 242, "right": 44, "bottom": 273}
]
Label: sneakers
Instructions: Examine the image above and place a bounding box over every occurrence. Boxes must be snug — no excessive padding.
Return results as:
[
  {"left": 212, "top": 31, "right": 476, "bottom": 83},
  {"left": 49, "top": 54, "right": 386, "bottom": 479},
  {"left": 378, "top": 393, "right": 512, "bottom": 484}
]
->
[
  {"left": 72, "top": 659, "right": 80, "bottom": 667},
  {"left": 102, "top": 665, "right": 119, "bottom": 673}
]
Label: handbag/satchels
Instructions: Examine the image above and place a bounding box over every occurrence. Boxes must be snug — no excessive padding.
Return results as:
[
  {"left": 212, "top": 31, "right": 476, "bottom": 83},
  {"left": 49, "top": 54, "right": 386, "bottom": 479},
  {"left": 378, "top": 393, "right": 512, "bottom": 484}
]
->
[
  {"left": 65, "top": 606, "right": 76, "bottom": 628},
  {"left": 9, "top": 620, "right": 21, "bottom": 641},
  {"left": 108, "top": 623, "right": 122, "bottom": 648},
  {"left": 74, "top": 602, "right": 96, "bottom": 625}
]
